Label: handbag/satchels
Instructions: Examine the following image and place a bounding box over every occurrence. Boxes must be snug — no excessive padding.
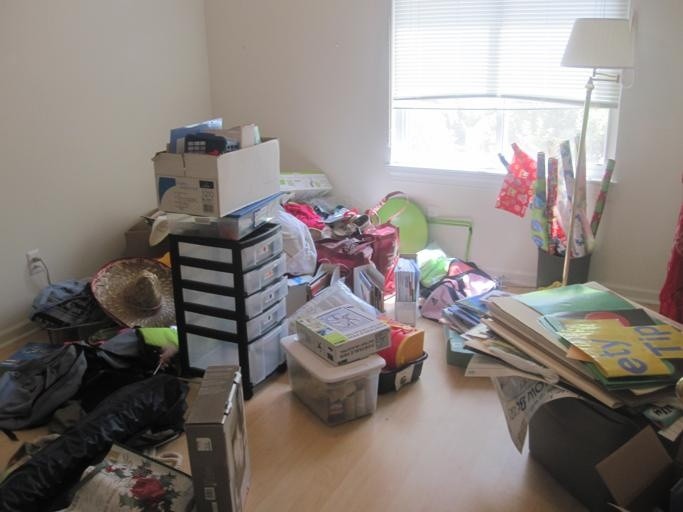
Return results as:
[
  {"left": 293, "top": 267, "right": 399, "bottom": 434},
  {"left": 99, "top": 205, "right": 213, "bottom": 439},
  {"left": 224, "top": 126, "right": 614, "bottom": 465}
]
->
[
  {"left": 0, "top": 372, "right": 184, "bottom": 512},
  {"left": 315, "top": 214, "right": 402, "bottom": 303},
  {"left": 417, "top": 256, "right": 501, "bottom": 325}
]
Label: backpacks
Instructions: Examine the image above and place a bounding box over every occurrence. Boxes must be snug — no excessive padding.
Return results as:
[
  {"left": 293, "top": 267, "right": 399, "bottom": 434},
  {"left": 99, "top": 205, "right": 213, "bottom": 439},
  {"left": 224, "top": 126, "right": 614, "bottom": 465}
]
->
[
  {"left": 28, "top": 278, "right": 114, "bottom": 335},
  {"left": 0, "top": 339, "right": 89, "bottom": 431}
]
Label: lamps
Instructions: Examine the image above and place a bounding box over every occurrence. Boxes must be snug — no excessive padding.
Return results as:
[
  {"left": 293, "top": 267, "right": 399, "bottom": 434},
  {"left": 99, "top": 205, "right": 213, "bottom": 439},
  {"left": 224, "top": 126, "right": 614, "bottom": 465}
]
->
[{"left": 561, "top": 17, "right": 635, "bottom": 293}]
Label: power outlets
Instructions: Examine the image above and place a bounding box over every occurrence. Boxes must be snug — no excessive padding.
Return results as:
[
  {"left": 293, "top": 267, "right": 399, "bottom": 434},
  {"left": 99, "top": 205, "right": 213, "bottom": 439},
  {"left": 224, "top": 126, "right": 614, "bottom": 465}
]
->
[{"left": 25, "top": 248, "right": 44, "bottom": 278}]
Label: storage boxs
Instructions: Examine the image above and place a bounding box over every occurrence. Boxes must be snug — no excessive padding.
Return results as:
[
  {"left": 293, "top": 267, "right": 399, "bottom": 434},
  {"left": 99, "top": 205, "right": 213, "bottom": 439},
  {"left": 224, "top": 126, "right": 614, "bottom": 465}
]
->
[
  {"left": 153, "top": 136, "right": 282, "bottom": 219},
  {"left": 295, "top": 303, "right": 394, "bottom": 367},
  {"left": 166, "top": 222, "right": 291, "bottom": 402},
  {"left": 158, "top": 193, "right": 283, "bottom": 242},
  {"left": 184, "top": 365, "right": 252, "bottom": 512},
  {"left": 520, "top": 376, "right": 682, "bottom": 511},
  {"left": 282, "top": 173, "right": 334, "bottom": 202},
  {"left": 288, "top": 271, "right": 330, "bottom": 316},
  {"left": 278, "top": 330, "right": 387, "bottom": 428}
]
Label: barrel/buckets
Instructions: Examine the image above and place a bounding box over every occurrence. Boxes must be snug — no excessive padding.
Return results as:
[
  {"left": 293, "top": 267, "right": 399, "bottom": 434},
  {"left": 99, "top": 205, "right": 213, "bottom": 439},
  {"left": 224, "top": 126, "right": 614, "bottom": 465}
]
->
[{"left": 537, "top": 247, "right": 590, "bottom": 287}]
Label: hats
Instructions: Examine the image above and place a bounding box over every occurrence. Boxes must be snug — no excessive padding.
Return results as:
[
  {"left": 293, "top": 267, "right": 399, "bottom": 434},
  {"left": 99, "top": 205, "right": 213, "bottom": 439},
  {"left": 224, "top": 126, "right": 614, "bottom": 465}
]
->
[{"left": 89, "top": 255, "right": 178, "bottom": 330}]
